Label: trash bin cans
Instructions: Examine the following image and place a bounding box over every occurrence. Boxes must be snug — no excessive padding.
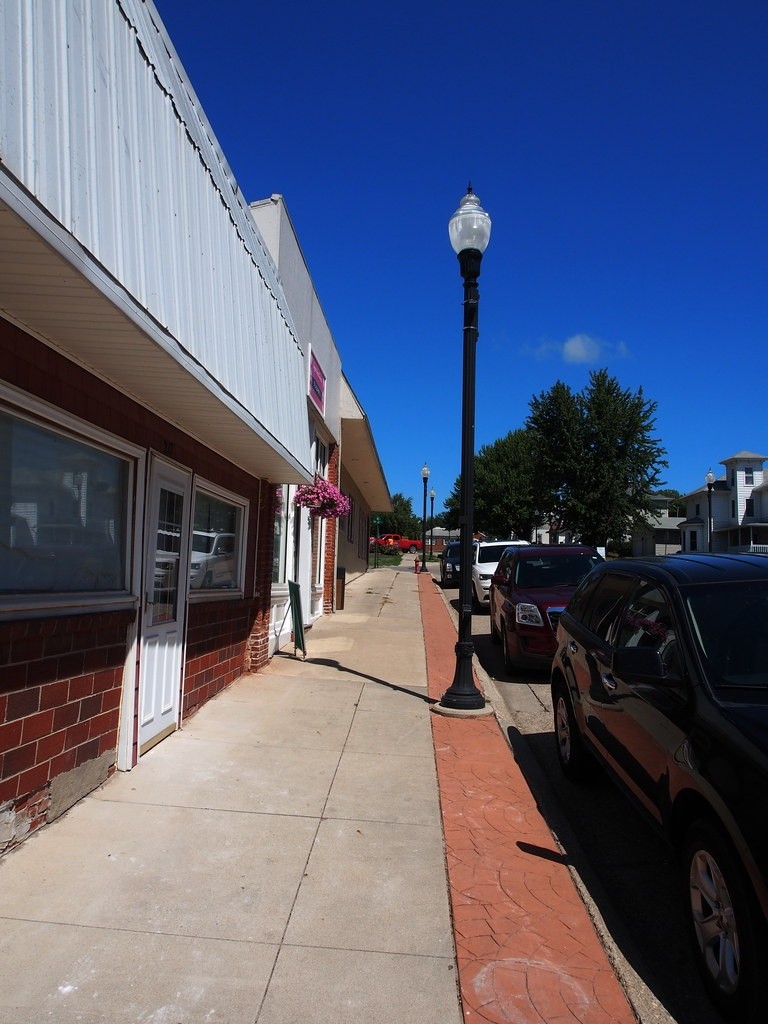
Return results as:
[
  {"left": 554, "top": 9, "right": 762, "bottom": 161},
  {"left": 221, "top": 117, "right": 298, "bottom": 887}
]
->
[{"left": 336, "top": 566, "right": 346, "bottom": 610}]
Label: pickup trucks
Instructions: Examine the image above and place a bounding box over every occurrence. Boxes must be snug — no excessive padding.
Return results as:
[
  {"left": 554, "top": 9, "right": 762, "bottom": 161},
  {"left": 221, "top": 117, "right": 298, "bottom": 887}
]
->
[{"left": 371, "top": 534, "right": 423, "bottom": 554}]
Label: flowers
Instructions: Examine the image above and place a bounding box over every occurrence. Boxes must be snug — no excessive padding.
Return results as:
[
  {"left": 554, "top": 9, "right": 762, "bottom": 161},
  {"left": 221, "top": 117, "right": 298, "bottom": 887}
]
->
[
  {"left": 292, "top": 474, "right": 350, "bottom": 520},
  {"left": 624, "top": 612, "right": 668, "bottom": 641}
]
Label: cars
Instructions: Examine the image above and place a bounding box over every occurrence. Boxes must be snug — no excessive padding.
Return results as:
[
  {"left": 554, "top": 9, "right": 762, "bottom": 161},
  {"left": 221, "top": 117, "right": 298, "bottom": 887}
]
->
[
  {"left": 437, "top": 542, "right": 463, "bottom": 587},
  {"left": 549, "top": 554, "right": 767, "bottom": 1016},
  {"left": 472, "top": 539, "right": 530, "bottom": 612}
]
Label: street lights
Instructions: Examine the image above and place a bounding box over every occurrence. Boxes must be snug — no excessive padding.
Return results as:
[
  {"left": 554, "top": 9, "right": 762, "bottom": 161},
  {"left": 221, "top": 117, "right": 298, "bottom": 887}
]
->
[
  {"left": 441, "top": 182, "right": 493, "bottom": 710},
  {"left": 420, "top": 462, "right": 430, "bottom": 572},
  {"left": 428, "top": 486, "right": 436, "bottom": 561}
]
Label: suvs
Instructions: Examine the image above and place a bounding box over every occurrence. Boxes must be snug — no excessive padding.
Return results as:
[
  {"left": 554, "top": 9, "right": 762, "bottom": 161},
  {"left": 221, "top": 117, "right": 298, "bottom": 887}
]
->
[{"left": 489, "top": 544, "right": 606, "bottom": 677}]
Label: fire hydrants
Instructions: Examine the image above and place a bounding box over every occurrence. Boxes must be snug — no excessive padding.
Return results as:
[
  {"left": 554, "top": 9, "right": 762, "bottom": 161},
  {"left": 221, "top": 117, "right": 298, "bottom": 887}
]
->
[{"left": 414, "top": 557, "right": 420, "bottom": 573}]
